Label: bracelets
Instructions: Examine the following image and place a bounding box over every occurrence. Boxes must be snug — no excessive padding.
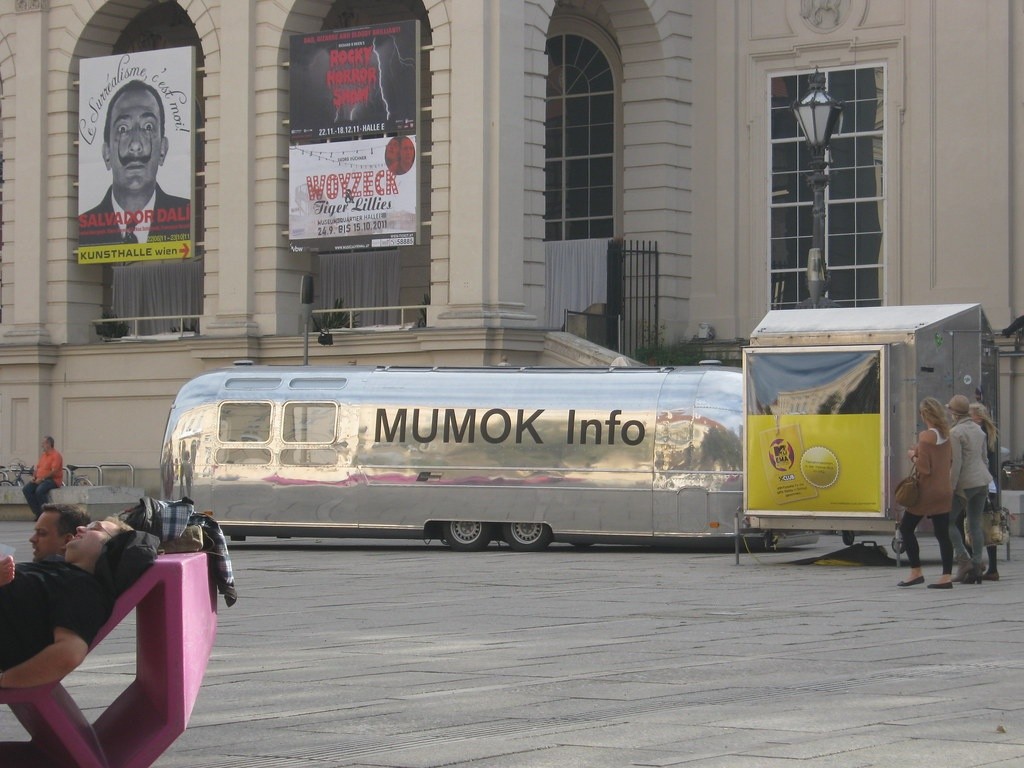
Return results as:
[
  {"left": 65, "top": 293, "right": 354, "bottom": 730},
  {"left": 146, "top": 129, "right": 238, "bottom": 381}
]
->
[{"left": 911, "top": 455, "right": 918, "bottom": 462}]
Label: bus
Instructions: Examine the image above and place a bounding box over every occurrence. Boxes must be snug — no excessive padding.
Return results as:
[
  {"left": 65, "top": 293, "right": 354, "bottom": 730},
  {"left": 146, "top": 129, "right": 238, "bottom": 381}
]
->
[{"left": 156, "top": 355, "right": 748, "bottom": 546}]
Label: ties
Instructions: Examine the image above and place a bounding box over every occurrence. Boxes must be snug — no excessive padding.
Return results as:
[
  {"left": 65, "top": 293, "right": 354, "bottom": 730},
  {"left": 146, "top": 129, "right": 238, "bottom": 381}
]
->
[{"left": 124, "top": 221, "right": 138, "bottom": 244}]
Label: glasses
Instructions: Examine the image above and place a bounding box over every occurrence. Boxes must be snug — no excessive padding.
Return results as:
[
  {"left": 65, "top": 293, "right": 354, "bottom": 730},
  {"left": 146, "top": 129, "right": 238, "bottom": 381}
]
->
[{"left": 87, "top": 520, "right": 113, "bottom": 539}]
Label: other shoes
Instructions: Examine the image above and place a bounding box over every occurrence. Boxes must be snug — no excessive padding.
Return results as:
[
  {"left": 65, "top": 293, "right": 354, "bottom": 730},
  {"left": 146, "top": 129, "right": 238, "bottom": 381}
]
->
[
  {"left": 926, "top": 582, "right": 953, "bottom": 589},
  {"left": 897, "top": 576, "right": 924, "bottom": 586},
  {"left": 982, "top": 571, "right": 999, "bottom": 581}
]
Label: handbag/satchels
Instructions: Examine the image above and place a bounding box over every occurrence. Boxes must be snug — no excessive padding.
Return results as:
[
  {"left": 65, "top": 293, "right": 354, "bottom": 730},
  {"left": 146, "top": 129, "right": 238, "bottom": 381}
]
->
[
  {"left": 963, "top": 494, "right": 1009, "bottom": 547},
  {"left": 157, "top": 523, "right": 203, "bottom": 555},
  {"left": 894, "top": 462, "right": 919, "bottom": 507}
]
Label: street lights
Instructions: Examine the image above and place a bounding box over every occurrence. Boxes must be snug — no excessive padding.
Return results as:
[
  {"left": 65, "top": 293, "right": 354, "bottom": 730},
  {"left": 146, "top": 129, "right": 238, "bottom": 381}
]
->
[{"left": 794, "top": 79, "right": 847, "bottom": 310}]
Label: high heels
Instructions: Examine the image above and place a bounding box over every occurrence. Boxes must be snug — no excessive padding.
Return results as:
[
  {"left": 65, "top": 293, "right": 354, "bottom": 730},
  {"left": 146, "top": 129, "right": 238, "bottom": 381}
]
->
[
  {"left": 960, "top": 563, "right": 983, "bottom": 584},
  {"left": 951, "top": 559, "right": 978, "bottom": 582}
]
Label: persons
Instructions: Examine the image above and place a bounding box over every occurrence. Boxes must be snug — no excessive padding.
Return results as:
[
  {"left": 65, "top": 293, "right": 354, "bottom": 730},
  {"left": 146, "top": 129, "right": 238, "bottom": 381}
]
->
[
  {"left": 22, "top": 436, "right": 64, "bottom": 522},
  {"left": 28, "top": 504, "right": 92, "bottom": 565},
  {"left": 968, "top": 403, "right": 1001, "bottom": 582},
  {"left": 1, "top": 516, "right": 136, "bottom": 688},
  {"left": 898, "top": 397, "right": 956, "bottom": 590},
  {"left": 78, "top": 81, "right": 190, "bottom": 244},
  {"left": 944, "top": 395, "right": 993, "bottom": 585}
]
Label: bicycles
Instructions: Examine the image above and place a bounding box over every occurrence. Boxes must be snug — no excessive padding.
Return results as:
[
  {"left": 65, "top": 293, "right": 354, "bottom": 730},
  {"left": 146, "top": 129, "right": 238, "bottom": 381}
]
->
[{"left": 0, "top": 461, "right": 95, "bottom": 489}]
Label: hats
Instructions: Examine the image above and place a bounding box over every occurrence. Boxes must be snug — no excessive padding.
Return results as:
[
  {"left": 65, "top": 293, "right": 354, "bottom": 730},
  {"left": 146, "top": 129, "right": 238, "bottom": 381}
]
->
[{"left": 944, "top": 394, "right": 972, "bottom": 416}]
